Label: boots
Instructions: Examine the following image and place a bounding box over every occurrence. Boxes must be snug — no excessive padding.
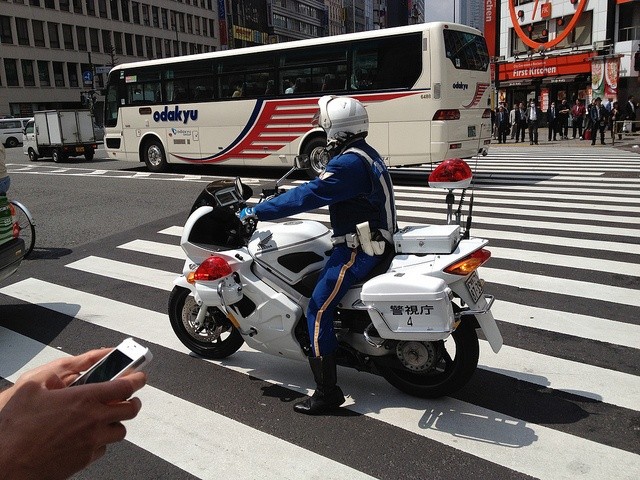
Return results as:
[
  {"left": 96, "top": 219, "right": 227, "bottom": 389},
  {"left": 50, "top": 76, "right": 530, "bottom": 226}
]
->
[{"left": 294, "top": 352, "right": 345, "bottom": 415}]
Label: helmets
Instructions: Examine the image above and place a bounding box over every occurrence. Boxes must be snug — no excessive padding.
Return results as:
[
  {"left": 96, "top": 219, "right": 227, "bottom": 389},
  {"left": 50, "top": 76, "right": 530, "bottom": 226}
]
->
[{"left": 311, "top": 94, "right": 369, "bottom": 150}]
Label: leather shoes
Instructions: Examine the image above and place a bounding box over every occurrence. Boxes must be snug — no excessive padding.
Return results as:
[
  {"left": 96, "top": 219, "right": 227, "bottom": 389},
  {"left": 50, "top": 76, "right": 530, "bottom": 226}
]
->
[
  {"left": 521, "top": 140, "right": 525, "bottom": 142},
  {"left": 535, "top": 142, "right": 538, "bottom": 145},
  {"left": 601, "top": 142, "right": 606, "bottom": 145},
  {"left": 591, "top": 142, "right": 596, "bottom": 145},
  {"left": 515, "top": 141, "right": 518, "bottom": 143},
  {"left": 530, "top": 142, "right": 533, "bottom": 145}
]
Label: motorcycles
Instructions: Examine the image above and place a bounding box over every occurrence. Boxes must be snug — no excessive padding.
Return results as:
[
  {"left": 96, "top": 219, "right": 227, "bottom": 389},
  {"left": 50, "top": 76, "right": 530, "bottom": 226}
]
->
[{"left": 168, "top": 122, "right": 503, "bottom": 399}]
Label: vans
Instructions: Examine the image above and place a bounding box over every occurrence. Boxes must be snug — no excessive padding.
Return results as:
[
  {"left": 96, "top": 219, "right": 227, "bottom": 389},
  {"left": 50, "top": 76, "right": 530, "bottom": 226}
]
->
[{"left": 0, "top": 117, "right": 34, "bottom": 148}]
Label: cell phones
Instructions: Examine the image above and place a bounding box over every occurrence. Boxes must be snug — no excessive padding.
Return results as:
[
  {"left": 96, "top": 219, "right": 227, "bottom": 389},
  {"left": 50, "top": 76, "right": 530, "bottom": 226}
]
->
[{"left": 63, "top": 338, "right": 153, "bottom": 390}]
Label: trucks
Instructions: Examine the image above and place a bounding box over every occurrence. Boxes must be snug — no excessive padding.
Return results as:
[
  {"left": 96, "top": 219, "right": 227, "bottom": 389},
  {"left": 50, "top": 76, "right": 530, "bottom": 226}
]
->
[{"left": 22, "top": 109, "right": 98, "bottom": 162}]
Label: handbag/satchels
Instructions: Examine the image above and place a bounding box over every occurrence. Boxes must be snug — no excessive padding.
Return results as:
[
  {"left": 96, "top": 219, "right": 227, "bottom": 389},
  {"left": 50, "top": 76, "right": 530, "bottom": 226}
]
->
[
  {"left": 585, "top": 129, "right": 591, "bottom": 140},
  {"left": 622, "top": 119, "right": 632, "bottom": 132}
]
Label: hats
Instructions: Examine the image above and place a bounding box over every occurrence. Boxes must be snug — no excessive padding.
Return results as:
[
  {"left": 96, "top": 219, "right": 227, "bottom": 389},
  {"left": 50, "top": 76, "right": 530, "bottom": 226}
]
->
[{"left": 595, "top": 97, "right": 602, "bottom": 102}]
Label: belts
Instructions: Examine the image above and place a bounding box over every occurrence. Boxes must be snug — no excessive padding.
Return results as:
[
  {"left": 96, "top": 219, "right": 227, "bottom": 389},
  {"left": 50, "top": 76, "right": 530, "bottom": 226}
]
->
[{"left": 330, "top": 231, "right": 374, "bottom": 246}]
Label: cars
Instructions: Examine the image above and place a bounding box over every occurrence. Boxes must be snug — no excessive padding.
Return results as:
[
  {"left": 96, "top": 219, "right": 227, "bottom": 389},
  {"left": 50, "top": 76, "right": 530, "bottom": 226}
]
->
[
  {"left": 1, "top": 194, "right": 26, "bottom": 283},
  {"left": 93, "top": 122, "right": 105, "bottom": 141}
]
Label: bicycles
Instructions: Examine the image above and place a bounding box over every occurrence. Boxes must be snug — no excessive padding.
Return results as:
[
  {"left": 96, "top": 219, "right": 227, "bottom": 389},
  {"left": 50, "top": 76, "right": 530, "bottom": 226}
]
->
[{"left": 0, "top": 192, "right": 37, "bottom": 258}]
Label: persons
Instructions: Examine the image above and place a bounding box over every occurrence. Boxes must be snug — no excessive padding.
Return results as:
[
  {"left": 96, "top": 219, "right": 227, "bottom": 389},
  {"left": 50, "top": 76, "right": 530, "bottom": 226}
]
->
[
  {"left": 237, "top": 94, "right": 399, "bottom": 417},
  {"left": 623, "top": 95, "right": 640, "bottom": 133},
  {"left": 509, "top": 104, "right": 519, "bottom": 139},
  {"left": 583, "top": 98, "right": 595, "bottom": 139},
  {"left": 0, "top": 138, "right": 11, "bottom": 195},
  {"left": 515, "top": 102, "right": 529, "bottom": 143},
  {"left": 496, "top": 106, "right": 508, "bottom": 144},
  {"left": 546, "top": 102, "right": 559, "bottom": 142},
  {"left": 609, "top": 102, "right": 623, "bottom": 140},
  {"left": 523, "top": 101, "right": 543, "bottom": 145},
  {"left": 571, "top": 99, "right": 586, "bottom": 140},
  {"left": 605, "top": 98, "right": 614, "bottom": 131},
  {"left": 557, "top": 98, "right": 570, "bottom": 141},
  {"left": 0, "top": 347, "right": 148, "bottom": 479},
  {"left": 590, "top": 97, "right": 609, "bottom": 145}
]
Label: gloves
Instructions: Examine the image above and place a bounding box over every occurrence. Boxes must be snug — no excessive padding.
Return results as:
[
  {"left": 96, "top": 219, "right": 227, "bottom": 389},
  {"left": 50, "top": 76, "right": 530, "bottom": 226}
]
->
[{"left": 240, "top": 206, "right": 259, "bottom": 225}]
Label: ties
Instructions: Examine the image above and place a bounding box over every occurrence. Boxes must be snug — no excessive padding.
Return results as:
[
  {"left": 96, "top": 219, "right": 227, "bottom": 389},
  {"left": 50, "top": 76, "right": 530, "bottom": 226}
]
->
[
  {"left": 553, "top": 108, "right": 554, "bottom": 117},
  {"left": 532, "top": 108, "right": 534, "bottom": 119}
]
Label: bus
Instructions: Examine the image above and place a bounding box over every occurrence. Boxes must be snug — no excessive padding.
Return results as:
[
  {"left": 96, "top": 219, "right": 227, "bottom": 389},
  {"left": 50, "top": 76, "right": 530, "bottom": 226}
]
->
[{"left": 87, "top": 21, "right": 493, "bottom": 173}]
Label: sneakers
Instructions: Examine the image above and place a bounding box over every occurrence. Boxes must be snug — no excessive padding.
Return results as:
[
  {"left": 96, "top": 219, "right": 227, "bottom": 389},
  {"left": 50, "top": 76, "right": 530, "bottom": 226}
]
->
[
  {"left": 570, "top": 137, "right": 576, "bottom": 139},
  {"left": 579, "top": 137, "right": 583, "bottom": 140},
  {"left": 558, "top": 136, "right": 563, "bottom": 140},
  {"left": 564, "top": 136, "right": 568, "bottom": 140}
]
